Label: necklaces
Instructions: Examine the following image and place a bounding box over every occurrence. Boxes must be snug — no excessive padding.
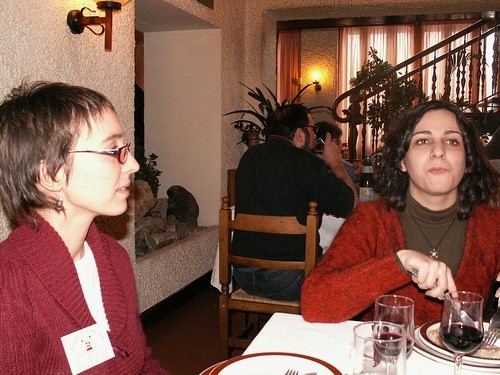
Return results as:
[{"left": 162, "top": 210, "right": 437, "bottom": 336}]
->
[{"left": 410, "top": 212, "right": 457, "bottom": 258}]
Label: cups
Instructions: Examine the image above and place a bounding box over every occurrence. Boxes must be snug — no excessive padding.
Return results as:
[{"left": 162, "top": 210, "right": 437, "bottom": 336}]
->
[
  {"left": 314, "top": 137, "right": 325, "bottom": 154},
  {"left": 352, "top": 321, "right": 408, "bottom": 375}
]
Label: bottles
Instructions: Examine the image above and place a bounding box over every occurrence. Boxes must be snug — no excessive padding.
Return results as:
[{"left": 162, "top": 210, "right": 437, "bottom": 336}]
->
[{"left": 359, "top": 156, "right": 376, "bottom": 203}]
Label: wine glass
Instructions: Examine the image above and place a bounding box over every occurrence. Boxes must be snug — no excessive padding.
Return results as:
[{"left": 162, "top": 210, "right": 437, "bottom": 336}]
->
[
  {"left": 373, "top": 294, "right": 415, "bottom": 375},
  {"left": 438, "top": 291, "right": 484, "bottom": 375}
]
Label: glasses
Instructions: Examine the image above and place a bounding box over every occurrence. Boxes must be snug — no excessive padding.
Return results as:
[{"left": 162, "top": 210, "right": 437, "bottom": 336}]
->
[
  {"left": 298, "top": 124, "right": 319, "bottom": 135},
  {"left": 51, "top": 143, "right": 131, "bottom": 165}
]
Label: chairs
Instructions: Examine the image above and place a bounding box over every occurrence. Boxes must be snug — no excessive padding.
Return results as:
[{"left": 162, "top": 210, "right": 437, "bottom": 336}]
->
[{"left": 218, "top": 196, "right": 320, "bottom": 362}]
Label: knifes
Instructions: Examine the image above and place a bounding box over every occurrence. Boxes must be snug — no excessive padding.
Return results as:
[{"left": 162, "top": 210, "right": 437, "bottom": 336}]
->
[{"left": 409, "top": 266, "right": 477, "bottom": 330}]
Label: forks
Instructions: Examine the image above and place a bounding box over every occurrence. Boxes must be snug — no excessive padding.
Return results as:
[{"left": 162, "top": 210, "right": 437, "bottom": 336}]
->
[{"left": 484, "top": 307, "right": 500, "bottom": 346}]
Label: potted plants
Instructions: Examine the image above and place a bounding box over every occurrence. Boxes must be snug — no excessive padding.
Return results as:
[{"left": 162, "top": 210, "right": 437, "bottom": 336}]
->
[{"left": 235, "top": 121, "right": 261, "bottom": 148}]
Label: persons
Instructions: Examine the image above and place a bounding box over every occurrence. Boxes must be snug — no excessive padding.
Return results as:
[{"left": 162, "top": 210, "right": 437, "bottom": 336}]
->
[
  {"left": 0, "top": 79, "right": 172, "bottom": 375},
  {"left": 231, "top": 104, "right": 359, "bottom": 302},
  {"left": 301, "top": 100, "right": 500, "bottom": 327}
]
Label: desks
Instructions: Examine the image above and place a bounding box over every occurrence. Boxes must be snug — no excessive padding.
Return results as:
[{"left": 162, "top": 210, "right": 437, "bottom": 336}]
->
[
  {"left": 241, "top": 312, "right": 500, "bottom": 375},
  {"left": 209, "top": 204, "right": 348, "bottom": 314}
]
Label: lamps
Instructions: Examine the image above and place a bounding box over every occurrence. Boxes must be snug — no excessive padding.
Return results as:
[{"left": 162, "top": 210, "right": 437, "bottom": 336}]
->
[
  {"left": 311, "top": 72, "right": 321, "bottom": 95},
  {"left": 66, "top": 0, "right": 132, "bottom": 52}
]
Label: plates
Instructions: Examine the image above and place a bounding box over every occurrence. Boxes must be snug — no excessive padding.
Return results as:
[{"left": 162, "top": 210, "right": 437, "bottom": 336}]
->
[
  {"left": 409, "top": 319, "right": 500, "bottom": 369},
  {"left": 199, "top": 353, "right": 341, "bottom": 375}
]
